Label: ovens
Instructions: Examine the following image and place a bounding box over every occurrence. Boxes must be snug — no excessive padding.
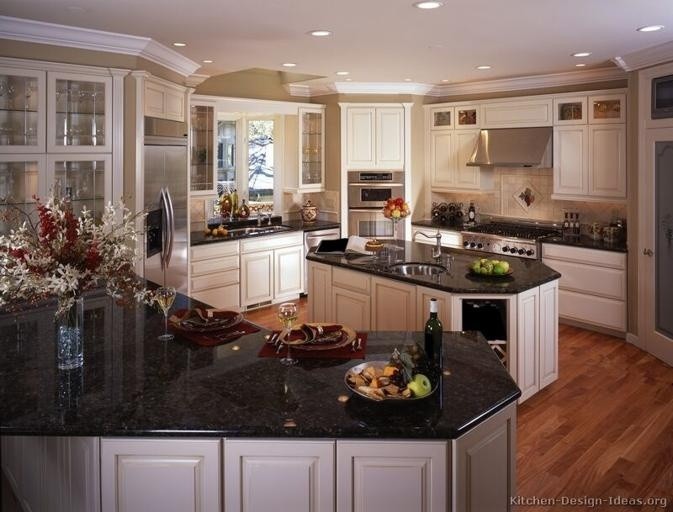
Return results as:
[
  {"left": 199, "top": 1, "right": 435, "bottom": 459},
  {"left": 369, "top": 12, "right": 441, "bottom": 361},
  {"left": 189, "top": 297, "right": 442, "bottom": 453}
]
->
[{"left": 347, "top": 171, "right": 405, "bottom": 240}]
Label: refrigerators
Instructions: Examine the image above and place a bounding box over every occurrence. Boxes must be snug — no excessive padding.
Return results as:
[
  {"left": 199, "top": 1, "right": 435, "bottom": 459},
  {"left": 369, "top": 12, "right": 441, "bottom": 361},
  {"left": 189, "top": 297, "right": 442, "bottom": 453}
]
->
[{"left": 143, "top": 117, "right": 187, "bottom": 297}]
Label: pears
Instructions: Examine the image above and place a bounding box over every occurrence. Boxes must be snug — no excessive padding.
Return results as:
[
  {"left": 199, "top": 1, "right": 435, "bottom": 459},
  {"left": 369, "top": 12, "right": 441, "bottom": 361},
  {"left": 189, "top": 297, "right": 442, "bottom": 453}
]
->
[{"left": 205, "top": 226, "right": 228, "bottom": 236}]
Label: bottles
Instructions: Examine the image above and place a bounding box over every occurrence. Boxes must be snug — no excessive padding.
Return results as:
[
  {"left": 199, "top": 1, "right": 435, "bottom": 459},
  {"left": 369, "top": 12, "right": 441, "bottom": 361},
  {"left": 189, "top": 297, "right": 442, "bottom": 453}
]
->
[
  {"left": 468, "top": 200, "right": 474, "bottom": 221},
  {"left": 424, "top": 298, "right": 443, "bottom": 370},
  {"left": 562, "top": 211, "right": 581, "bottom": 236},
  {"left": 300, "top": 199, "right": 318, "bottom": 224},
  {"left": 431, "top": 204, "right": 465, "bottom": 224},
  {"left": 213, "top": 188, "right": 249, "bottom": 220}
]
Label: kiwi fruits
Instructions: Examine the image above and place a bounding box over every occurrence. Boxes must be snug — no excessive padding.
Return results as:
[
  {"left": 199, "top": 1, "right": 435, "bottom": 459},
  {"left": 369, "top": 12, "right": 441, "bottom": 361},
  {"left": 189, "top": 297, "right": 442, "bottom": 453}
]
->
[
  {"left": 409, "top": 344, "right": 424, "bottom": 361},
  {"left": 367, "top": 241, "right": 381, "bottom": 245}
]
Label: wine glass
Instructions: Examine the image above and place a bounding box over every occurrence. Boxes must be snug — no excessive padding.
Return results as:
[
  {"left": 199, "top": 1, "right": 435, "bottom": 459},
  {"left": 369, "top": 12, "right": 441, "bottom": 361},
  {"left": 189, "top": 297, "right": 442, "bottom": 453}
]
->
[
  {"left": 278, "top": 301, "right": 298, "bottom": 365},
  {"left": 0, "top": 77, "right": 104, "bottom": 146},
  {"left": 155, "top": 285, "right": 175, "bottom": 342},
  {"left": 303, "top": 117, "right": 321, "bottom": 184}
]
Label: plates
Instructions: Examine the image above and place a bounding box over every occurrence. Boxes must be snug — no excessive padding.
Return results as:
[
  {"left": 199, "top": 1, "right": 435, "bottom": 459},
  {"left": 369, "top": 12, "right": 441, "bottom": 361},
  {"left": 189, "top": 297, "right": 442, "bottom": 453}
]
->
[
  {"left": 169, "top": 308, "right": 243, "bottom": 334},
  {"left": 341, "top": 358, "right": 439, "bottom": 405},
  {"left": 466, "top": 263, "right": 514, "bottom": 278},
  {"left": 280, "top": 322, "right": 355, "bottom": 351}
]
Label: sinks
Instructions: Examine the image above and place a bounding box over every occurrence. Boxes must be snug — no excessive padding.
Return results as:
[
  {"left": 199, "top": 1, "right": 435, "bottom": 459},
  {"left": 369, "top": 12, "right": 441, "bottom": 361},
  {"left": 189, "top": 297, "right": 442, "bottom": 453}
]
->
[
  {"left": 388, "top": 261, "right": 447, "bottom": 276},
  {"left": 247, "top": 225, "right": 292, "bottom": 236}
]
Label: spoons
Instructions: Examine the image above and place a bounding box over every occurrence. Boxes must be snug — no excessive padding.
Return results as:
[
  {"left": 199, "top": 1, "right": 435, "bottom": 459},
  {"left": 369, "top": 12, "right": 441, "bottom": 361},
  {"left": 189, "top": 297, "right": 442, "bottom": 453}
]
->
[{"left": 267, "top": 333, "right": 277, "bottom": 344}]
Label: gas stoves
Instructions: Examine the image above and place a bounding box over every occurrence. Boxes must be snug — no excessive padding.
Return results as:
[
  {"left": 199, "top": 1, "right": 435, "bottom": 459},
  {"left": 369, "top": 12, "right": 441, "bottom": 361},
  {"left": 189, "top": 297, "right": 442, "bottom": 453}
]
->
[{"left": 463, "top": 220, "right": 560, "bottom": 258}]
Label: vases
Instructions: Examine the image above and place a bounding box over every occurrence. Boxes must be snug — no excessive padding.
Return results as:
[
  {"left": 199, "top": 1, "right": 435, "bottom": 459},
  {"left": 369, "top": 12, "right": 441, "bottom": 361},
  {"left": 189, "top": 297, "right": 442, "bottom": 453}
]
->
[
  {"left": 387, "top": 216, "right": 403, "bottom": 240},
  {"left": 52, "top": 293, "right": 84, "bottom": 374}
]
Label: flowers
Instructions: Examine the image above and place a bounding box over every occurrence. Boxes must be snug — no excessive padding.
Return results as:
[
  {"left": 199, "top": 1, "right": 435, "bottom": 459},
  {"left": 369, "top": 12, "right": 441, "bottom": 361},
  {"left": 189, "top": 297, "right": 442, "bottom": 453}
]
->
[
  {"left": 380, "top": 197, "right": 412, "bottom": 223},
  {"left": 0, "top": 189, "right": 165, "bottom": 342}
]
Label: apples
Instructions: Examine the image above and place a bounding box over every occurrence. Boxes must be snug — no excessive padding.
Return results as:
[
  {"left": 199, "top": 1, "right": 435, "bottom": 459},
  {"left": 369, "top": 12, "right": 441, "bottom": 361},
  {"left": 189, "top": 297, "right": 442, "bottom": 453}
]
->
[
  {"left": 407, "top": 374, "right": 430, "bottom": 398},
  {"left": 384, "top": 198, "right": 408, "bottom": 218}
]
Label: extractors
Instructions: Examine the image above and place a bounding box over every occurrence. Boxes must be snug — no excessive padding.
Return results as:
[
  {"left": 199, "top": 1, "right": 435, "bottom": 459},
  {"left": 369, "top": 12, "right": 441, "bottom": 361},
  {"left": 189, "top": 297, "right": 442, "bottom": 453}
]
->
[{"left": 465, "top": 128, "right": 552, "bottom": 168}]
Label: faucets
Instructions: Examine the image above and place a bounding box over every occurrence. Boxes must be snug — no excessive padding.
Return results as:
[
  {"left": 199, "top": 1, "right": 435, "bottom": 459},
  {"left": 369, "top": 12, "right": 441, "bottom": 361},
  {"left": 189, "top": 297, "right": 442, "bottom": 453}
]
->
[
  {"left": 257, "top": 207, "right": 266, "bottom": 226},
  {"left": 412, "top": 230, "right": 442, "bottom": 258}
]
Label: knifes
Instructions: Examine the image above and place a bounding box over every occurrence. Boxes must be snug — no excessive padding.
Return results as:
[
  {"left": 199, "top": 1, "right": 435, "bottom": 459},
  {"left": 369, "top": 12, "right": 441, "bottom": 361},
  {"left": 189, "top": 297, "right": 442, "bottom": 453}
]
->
[{"left": 349, "top": 338, "right": 363, "bottom": 353}]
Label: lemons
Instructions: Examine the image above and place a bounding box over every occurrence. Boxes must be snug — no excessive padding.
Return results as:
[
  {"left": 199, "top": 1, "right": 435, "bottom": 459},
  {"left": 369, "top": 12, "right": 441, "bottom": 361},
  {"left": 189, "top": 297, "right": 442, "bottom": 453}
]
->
[{"left": 468, "top": 258, "right": 509, "bottom": 274}]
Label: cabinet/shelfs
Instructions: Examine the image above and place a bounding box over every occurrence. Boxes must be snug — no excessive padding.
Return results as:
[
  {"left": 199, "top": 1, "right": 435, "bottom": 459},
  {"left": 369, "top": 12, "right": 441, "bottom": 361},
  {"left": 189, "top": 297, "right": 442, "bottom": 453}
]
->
[
  {"left": 340, "top": 101, "right": 412, "bottom": 173},
  {"left": 303, "top": 224, "right": 340, "bottom": 241},
  {"left": 0, "top": 56, "right": 122, "bottom": 239},
  {"left": 450, "top": 288, "right": 517, "bottom": 402},
  {"left": 330, "top": 263, "right": 372, "bottom": 332},
  {"left": 540, "top": 242, "right": 629, "bottom": 339},
  {"left": 305, "top": 258, "right": 329, "bottom": 330},
  {"left": 297, "top": 102, "right": 326, "bottom": 194},
  {"left": 190, "top": 238, "right": 240, "bottom": 318},
  {"left": 370, "top": 273, "right": 415, "bottom": 333},
  {"left": 416, "top": 279, "right": 450, "bottom": 331},
  {"left": 101, "top": 434, "right": 336, "bottom": 510},
  {"left": 141, "top": 72, "right": 185, "bottom": 122},
  {"left": 497, "top": 274, "right": 560, "bottom": 403},
  {"left": 550, "top": 123, "right": 629, "bottom": 206},
  {"left": 240, "top": 230, "right": 306, "bottom": 312},
  {"left": 187, "top": 96, "right": 217, "bottom": 199},
  {"left": 424, "top": 101, "right": 481, "bottom": 129},
  {"left": 451, "top": 393, "right": 516, "bottom": 512},
  {"left": 303, "top": 239, "right": 304, "bottom": 245},
  {"left": 426, "top": 129, "right": 481, "bottom": 196},
  {"left": 335, "top": 438, "right": 451, "bottom": 511},
  {"left": 553, "top": 86, "right": 629, "bottom": 123}
]
